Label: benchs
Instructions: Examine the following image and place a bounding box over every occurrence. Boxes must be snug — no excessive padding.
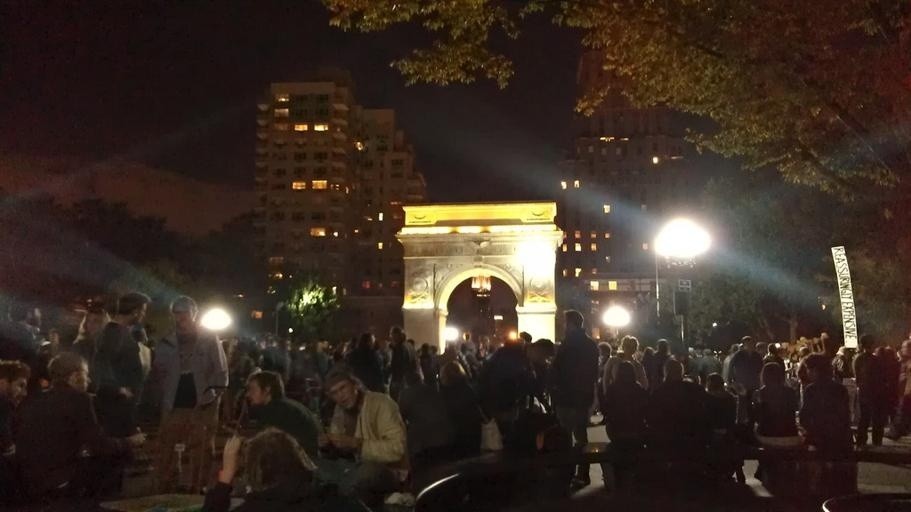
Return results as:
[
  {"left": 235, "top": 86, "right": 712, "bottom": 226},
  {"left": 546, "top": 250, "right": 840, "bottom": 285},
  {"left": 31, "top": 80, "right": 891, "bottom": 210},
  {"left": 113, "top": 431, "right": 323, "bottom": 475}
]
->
[
  {"left": 577, "top": 443, "right": 736, "bottom": 489},
  {"left": 736, "top": 437, "right": 910, "bottom": 494}
]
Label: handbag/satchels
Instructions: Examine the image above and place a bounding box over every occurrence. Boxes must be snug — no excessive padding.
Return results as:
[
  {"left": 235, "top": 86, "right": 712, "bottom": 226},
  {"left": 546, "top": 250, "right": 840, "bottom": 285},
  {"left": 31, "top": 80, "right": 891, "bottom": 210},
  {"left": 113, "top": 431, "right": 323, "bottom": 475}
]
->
[{"left": 479, "top": 418, "right": 503, "bottom": 453}]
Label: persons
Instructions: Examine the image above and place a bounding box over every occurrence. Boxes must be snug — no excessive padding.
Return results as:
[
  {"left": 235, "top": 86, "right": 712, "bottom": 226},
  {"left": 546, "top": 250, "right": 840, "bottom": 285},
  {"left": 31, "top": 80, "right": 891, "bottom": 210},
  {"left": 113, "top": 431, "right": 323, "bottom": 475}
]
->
[
  {"left": 0, "top": 291, "right": 232, "bottom": 511},
  {"left": 550, "top": 310, "right": 598, "bottom": 489},
  {"left": 210, "top": 333, "right": 561, "bottom": 511},
  {"left": 588, "top": 335, "right": 911, "bottom": 499}
]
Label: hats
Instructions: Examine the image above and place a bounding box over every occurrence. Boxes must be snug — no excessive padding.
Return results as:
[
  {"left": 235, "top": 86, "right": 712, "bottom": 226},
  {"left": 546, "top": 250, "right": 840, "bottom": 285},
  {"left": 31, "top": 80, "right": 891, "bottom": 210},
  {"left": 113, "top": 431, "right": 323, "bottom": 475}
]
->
[
  {"left": 322, "top": 361, "right": 354, "bottom": 394},
  {"left": 119, "top": 294, "right": 150, "bottom": 312},
  {"left": 172, "top": 295, "right": 195, "bottom": 312}
]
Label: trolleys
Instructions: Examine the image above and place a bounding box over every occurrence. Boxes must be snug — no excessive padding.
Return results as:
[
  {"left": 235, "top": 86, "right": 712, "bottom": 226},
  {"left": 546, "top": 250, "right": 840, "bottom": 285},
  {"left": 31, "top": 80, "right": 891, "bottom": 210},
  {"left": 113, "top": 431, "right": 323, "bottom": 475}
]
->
[{"left": 172, "top": 384, "right": 251, "bottom": 488}]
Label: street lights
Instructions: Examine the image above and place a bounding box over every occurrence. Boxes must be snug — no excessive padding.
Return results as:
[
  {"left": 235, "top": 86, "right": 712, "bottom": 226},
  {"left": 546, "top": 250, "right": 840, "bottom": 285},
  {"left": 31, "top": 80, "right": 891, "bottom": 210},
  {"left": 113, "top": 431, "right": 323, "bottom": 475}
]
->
[
  {"left": 702, "top": 322, "right": 719, "bottom": 346},
  {"left": 275, "top": 301, "right": 284, "bottom": 337},
  {"left": 653, "top": 216, "right": 714, "bottom": 365}
]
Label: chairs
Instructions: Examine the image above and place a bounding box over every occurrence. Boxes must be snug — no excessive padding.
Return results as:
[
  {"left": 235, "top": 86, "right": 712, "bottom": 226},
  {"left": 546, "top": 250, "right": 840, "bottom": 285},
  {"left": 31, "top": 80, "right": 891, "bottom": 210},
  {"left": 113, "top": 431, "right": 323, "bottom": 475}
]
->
[{"left": 412, "top": 466, "right": 498, "bottom": 512}]
